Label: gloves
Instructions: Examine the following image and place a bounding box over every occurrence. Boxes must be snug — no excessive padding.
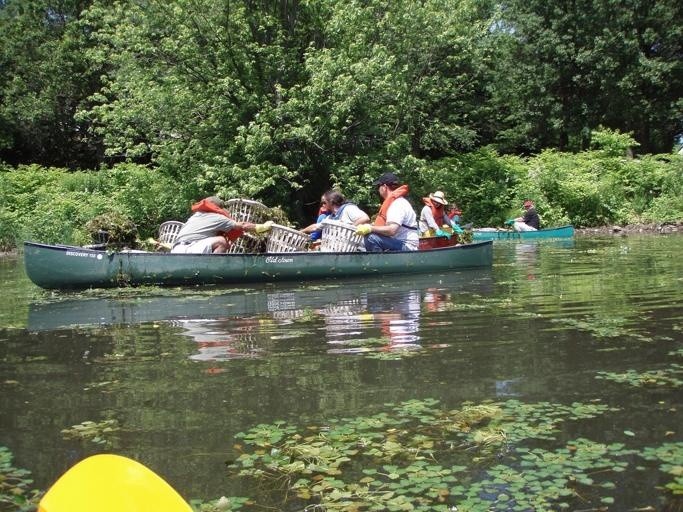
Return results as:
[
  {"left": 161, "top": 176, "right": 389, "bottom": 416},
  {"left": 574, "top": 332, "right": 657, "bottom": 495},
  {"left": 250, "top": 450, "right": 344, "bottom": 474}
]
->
[
  {"left": 451, "top": 224, "right": 463, "bottom": 234},
  {"left": 435, "top": 230, "right": 451, "bottom": 239},
  {"left": 504, "top": 220, "right": 514, "bottom": 226}
]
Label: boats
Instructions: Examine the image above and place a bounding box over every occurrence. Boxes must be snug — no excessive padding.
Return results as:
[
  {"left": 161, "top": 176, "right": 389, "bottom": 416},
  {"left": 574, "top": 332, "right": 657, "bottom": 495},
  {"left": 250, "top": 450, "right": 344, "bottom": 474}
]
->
[
  {"left": 467, "top": 225, "right": 577, "bottom": 241},
  {"left": 14, "top": 234, "right": 499, "bottom": 290},
  {"left": 14, "top": 272, "right": 496, "bottom": 335},
  {"left": 35, "top": 448, "right": 195, "bottom": 512}
]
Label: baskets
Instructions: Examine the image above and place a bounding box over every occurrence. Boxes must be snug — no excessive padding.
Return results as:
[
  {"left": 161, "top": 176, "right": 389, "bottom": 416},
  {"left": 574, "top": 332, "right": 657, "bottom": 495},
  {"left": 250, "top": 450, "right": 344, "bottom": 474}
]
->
[
  {"left": 159, "top": 220, "right": 184, "bottom": 244},
  {"left": 224, "top": 199, "right": 367, "bottom": 253}
]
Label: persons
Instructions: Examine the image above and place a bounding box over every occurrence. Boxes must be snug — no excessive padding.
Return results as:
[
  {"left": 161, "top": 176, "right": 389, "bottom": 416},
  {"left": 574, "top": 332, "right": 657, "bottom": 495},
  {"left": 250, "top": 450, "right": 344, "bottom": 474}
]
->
[
  {"left": 300, "top": 190, "right": 370, "bottom": 252},
  {"left": 448, "top": 204, "right": 462, "bottom": 227},
  {"left": 512, "top": 200, "right": 539, "bottom": 231},
  {"left": 364, "top": 172, "right": 420, "bottom": 252},
  {"left": 418, "top": 191, "right": 464, "bottom": 237},
  {"left": 171, "top": 196, "right": 274, "bottom": 254}
]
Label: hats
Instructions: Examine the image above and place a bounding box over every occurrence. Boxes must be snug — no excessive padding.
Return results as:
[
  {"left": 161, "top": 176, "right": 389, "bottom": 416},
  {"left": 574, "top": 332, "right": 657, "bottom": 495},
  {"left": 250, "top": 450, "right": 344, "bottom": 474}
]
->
[
  {"left": 372, "top": 173, "right": 399, "bottom": 185},
  {"left": 429, "top": 191, "right": 448, "bottom": 205},
  {"left": 523, "top": 201, "right": 532, "bottom": 207}
]
